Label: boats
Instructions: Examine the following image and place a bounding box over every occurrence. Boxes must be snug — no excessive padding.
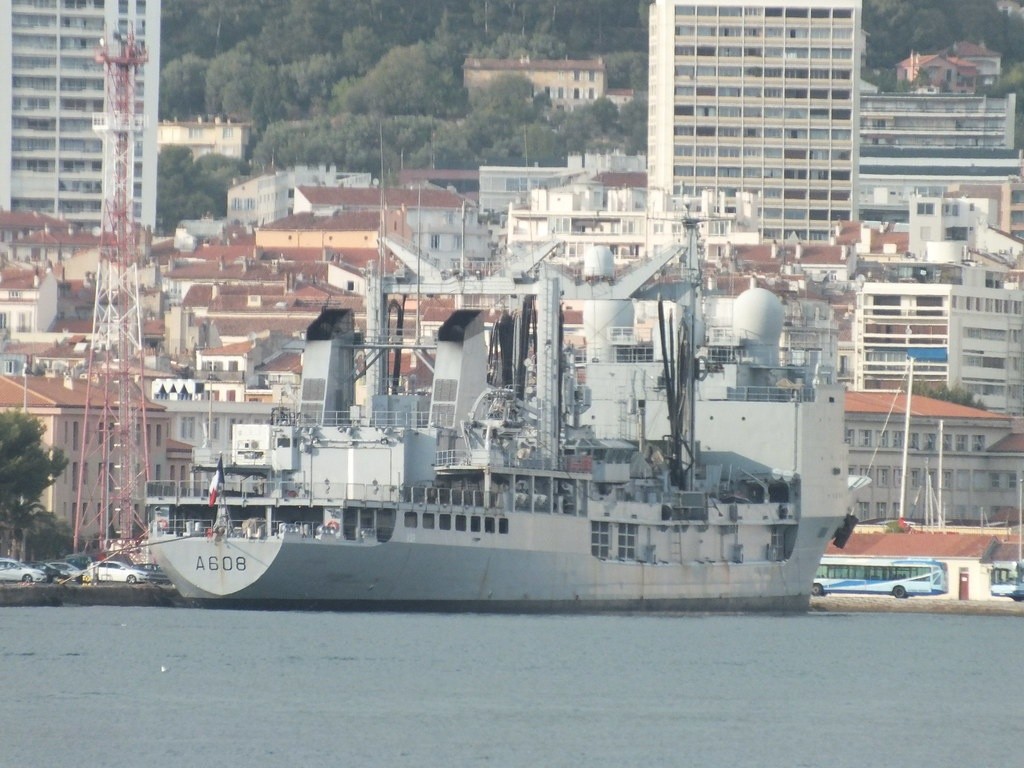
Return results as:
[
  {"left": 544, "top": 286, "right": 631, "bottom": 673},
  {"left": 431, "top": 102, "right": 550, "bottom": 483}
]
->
[{"left": 146, "top": 198, "right": 874, "bottom": 615}]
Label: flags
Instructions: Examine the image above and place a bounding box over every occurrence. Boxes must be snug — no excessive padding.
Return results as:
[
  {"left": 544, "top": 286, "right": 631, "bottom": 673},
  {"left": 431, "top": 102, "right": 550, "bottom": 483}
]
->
[{"left": 209, "top": 451, "right": 225, "bottom": 510}]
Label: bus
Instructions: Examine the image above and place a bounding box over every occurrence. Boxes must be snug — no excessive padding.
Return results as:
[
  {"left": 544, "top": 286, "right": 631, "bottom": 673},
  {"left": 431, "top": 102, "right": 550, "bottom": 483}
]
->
[
  {"left": 989, "top": 563, "right": 1024, "bottom": 601},
  {"left": 812, "top": 553, "right": 949, "bottom": 598}
]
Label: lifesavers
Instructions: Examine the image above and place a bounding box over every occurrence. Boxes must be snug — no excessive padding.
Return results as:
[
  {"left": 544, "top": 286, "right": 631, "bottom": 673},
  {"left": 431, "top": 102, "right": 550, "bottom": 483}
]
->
[
  {"left": 158, "top": 519, "right": 168, "bottom": 529},
  {"left": 327, "top": 520, "right": 339, "bottom": 529}
]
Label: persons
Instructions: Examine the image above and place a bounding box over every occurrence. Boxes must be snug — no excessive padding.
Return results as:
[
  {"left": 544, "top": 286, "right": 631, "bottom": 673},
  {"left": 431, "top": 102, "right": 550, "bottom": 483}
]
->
[{"left": 10, "top": 563, "right": 15, "bottom": 568}]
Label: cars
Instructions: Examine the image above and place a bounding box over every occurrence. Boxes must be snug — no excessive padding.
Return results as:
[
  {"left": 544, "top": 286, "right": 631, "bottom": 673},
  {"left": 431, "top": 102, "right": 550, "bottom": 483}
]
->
[
  {"left": 0, "top": 558, "right": 83, "bottom": 583},
  {"left": 88, "top": 560, "right": 172, "bottom": 584}
]
